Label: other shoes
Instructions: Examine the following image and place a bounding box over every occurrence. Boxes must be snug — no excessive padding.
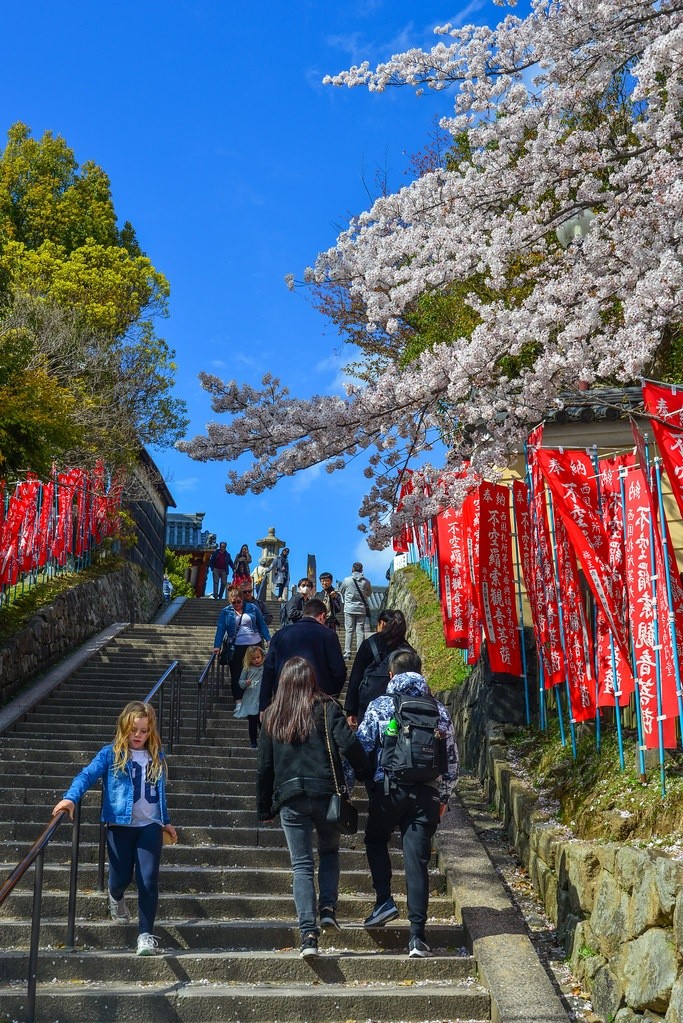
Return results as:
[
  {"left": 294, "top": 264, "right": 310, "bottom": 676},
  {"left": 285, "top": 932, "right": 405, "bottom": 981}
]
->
[{"left": 343, "top": 652, "right": 351, "bottom": 661}]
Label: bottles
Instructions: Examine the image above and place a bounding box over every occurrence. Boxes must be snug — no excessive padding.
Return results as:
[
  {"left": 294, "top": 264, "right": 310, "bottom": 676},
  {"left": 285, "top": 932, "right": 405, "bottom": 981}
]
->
[{"left": 385, "top": 718, "right": 399, "bottom": 737}]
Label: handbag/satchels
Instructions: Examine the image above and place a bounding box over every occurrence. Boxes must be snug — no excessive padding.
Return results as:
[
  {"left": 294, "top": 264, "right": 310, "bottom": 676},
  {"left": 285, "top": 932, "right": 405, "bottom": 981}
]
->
[
  {"left": 219, "top": 638, "right": 237, "bottom": 665},
  {"left": 366, "top": 603, "right": 372, "bottom": 618},
  {"left": 325, "top": 793, "right": 359, "bottom": 835}
]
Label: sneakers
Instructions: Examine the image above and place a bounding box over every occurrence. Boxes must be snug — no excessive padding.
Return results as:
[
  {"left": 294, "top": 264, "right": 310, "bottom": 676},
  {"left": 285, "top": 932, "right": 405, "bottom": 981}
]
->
[
  {"left": 364, "top": 895, "right": 400, "bottom": 927},
  {"left": 136, "top": 932, "right": 157, "bottom": 956},
  {"left": 408, "top": 935, "right": 434, "bottom": 957},
  {"left": 319, "top": 906, "right": 342, "bottom": 936},
  {"left": 298, "top": 936, "right": 319, "bottom": 959},
  {"left": 108, "top": 886, "right": 130, "bottom": 924}
]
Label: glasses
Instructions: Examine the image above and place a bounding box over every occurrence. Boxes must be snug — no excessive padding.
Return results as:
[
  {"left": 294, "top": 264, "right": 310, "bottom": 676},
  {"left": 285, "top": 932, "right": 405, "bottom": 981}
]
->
[
  {"left": 230, "top": 599, "right": 242, "bottom": 605},
  {"left": 302, "top": 584, "right": 311, "bottom": 588},
  {"left": 242, "top": 589, "right": 252, "bottom": 594}
]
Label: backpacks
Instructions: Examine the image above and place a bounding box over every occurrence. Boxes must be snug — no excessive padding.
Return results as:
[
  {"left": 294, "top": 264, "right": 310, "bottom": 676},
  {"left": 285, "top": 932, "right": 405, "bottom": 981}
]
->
[
  {"left": 381, "top": 691, "right": 449, "bottom": 796},
  {"left": 280, "top": 594, "right": 301, "bottom": 630},
  {"left": 357, "top": 638, "right": 392, "bottom": 711}
]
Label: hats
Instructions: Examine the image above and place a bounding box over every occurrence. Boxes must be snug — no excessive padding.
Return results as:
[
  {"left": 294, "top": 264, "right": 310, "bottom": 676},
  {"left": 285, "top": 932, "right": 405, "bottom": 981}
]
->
[{"left": 353, "top": 562, "right": 363, "bottom": 568}]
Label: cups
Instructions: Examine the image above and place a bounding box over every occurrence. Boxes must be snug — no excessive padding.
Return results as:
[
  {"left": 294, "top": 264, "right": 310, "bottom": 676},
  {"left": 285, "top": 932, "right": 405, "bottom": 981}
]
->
[{"left": 163, "top": 831, "right": 178, "bottom": 845}]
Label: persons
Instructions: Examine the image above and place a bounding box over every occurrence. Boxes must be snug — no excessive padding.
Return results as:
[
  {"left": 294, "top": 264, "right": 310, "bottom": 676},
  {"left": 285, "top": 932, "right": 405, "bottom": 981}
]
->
[
  {"left": 338, "top": 562, "right": 372, "bottom": 660},
  {"left": 266, "top": 547, "right": 291, "bottom": 601},
  {"left": 313, "top": 572, "right": 344, "bottom": 635},
  {"left": 338, "top": 648, "right": 460, "bottom": 959},
  {"left": 385, "top": 550, "right": 410, "bottom": 581},
  {"left": 232, "top": 645, "right": 269, "bottom": 749},
  {"left": 238, "top": 580, "right": 273, "bottom": 651},
  {"left": 250, "top": 560, "right": 270, "bottom": 603},
  {"left": 209, "top": 541, "right": 235, "bottom": 599},
  {"left": 258, "top": 598, "right": 348, "bottom": 727},
  {"left": 212, "top": 585, "right": 273, "bottom": 713},
  {"left": 51, "top": 700, "right": 177, "bottom": 956},
  {"left": 343, "top": 608, "right": 419, "bottom": 777},
  {"left": 231, "top": 544, "right": 253, "bottom": 597},
  {"left": 284, "top": 578, "right": 314, "bottom": 628},
  {"left": 255, "top": 656, "right": 378, "bottom": 959}
]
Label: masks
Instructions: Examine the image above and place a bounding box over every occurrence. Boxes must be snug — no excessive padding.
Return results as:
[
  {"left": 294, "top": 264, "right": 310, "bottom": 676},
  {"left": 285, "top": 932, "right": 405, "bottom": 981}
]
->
[{"left": 299, "top": 586, "right": 311, "bottom": 595}]
